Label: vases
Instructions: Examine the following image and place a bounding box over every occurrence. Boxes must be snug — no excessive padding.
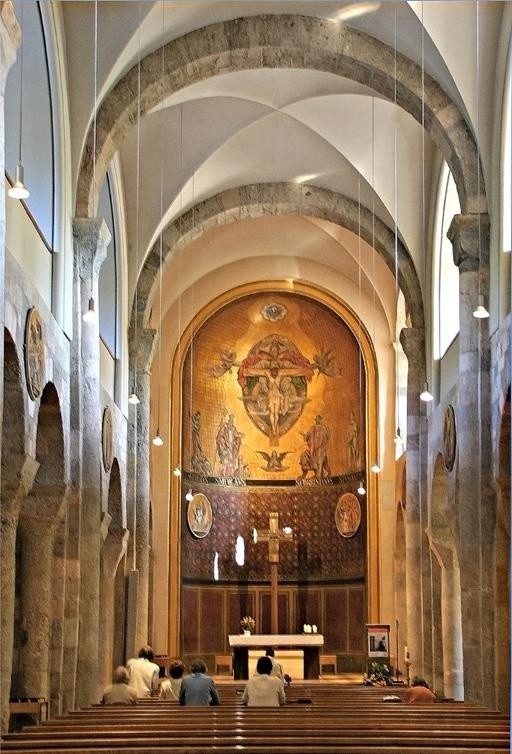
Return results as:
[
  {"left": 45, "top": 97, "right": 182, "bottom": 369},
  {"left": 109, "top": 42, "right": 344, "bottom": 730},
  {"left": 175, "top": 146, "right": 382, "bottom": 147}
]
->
[{"left": 244, "top": 629, "right": 250, "bottom": 636}]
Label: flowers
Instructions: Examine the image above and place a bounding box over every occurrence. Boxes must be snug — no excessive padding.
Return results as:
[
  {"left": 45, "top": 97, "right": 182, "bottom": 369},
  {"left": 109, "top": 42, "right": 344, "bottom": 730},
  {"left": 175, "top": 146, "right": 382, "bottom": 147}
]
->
[{"left": 240, "top": 616, "right": 256, "bottom": 632}]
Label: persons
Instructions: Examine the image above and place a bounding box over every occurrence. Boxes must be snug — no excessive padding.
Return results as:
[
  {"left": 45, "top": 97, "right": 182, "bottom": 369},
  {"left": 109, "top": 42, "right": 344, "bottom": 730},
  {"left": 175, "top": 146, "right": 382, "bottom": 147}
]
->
[
  {"left": 240, "top": 656, "right": 287, "bottom": 707},
  {"left": 159, "top": 658, "right": 185, "bottom": 701},
  {"left": 188, "top": 349, "right": 358, "bottom": 480},
  {"left": 125, "top": 645, "right": 161, "bottom": 700},
  {"left": 102, "top": 664, "right": 136, "bottom": 703},
  {"left": 157, "top": 666, "right": 170, "bottom": 690},
  {"left": 179, "top": 658, "right": 220, "bottom": 706},
  {"left": 402, "top": 678, "right": 437, "bottom": 704},
  {"left": 252, "top": 670, "right": 285, "bottom": 684},
  {"left": 379, "top": 635, "right": 387, "bottom": 651},
  {"left": 254, "top": 648, "right": 283, "bottom": 674}
]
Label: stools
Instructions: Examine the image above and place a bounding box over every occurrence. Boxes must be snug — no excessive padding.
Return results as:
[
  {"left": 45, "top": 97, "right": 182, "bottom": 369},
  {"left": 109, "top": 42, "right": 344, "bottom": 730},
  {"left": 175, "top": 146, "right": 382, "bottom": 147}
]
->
[
  {"left": 214, "top": 655, "right": 233, "bottom": 675},
  {"left": 319, "top": 655, "right": 337, "bottom": 675}
]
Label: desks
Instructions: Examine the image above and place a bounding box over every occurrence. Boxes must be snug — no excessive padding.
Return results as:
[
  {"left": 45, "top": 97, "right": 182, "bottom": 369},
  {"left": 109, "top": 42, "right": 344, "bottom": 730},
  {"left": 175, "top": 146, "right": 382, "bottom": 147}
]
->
[{"left": 228, "top": 634, "right": 324, "bottom": 680}]
